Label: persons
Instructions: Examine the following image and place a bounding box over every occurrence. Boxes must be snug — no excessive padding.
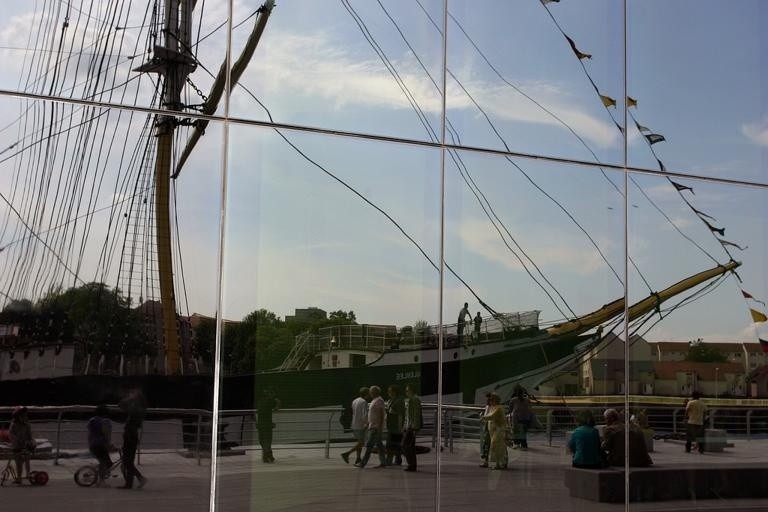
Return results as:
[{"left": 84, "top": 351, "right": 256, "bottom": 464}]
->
[
  {"left": 256, "top": 388, "right": 279, "bottom": 463},
  {"left": 339, "top": 383, "right": 533, "bottom": 472},
  {"left": 473, "top": 312, "right": 484, "bottom": 335},
  {"left": 118, "top": 381, "right": 147, "bottom": 489},
  {"left": 457, "top": 303, "right": 472, "bottom": 336},
  {"left": 9, "top": 404, "right": 35, "bottom": 484},
  {"left": 566, "top": 391, "right": 709, "bottom": 470},
  {"left": 86, "top": 402, "right": 113, "bottom": 468}
]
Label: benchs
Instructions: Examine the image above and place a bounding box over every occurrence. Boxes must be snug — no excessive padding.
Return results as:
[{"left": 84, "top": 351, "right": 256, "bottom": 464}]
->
[{"left": 561, "top": 461, "right": 767, "bottom": 503}]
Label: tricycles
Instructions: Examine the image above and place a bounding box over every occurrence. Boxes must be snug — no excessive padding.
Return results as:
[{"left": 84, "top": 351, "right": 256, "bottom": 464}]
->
[{"left": 0, "top": 448, "right": 51, "bottom": 488}]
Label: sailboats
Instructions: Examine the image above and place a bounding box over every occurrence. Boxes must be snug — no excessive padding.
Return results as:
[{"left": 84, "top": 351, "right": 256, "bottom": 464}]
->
[{"left": 0, "top": 0, "right": 743, "bottom": 453}]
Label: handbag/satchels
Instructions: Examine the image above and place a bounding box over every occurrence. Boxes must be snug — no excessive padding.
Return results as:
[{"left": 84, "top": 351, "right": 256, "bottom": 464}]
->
[{"left": 339, "top": 401, "right": 352, "bottom": 428}]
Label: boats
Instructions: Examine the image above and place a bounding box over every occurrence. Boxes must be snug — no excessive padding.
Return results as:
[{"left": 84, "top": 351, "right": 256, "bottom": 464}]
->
[{"left": 0, "top": 436, "right": 89, "bottom": 457}]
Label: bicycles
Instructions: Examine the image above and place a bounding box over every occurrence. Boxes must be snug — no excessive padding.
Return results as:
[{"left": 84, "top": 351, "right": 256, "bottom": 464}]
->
[{"left": 70, "top": 442, "right": 126, "bottom": 485}]
